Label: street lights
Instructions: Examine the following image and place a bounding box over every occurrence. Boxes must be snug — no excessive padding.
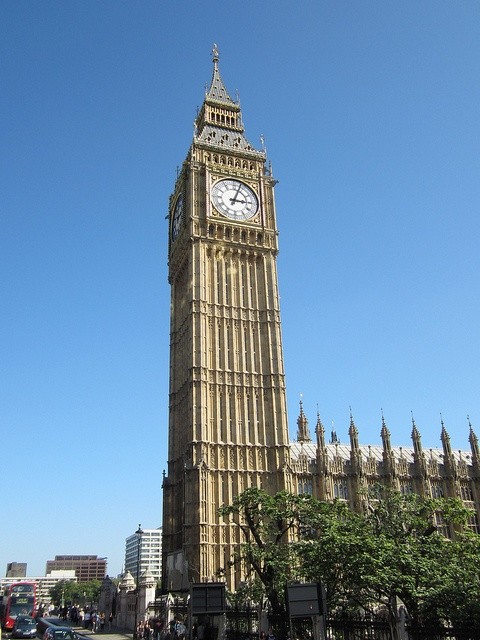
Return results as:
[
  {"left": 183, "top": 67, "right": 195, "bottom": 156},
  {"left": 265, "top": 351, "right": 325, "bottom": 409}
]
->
[{"left": 133, "top": 525, "right": 144, "bottom": 640}]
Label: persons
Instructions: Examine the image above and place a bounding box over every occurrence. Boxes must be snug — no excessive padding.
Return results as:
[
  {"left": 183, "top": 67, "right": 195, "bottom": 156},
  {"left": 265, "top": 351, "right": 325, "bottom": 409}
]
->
[
  {"left": 133, "top": 617, "right": 277, "bottom": 639},
  {"left": 37, "top": 601, "right": 113, "bottom": 636}
]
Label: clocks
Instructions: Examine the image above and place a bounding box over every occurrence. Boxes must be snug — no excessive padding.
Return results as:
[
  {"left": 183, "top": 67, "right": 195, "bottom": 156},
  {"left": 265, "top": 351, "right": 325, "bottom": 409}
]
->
[
  {"left": 171, "top": 194, "right": 183, "bottom": 242},
  {"left": 210, "top": 177, "right": 259, "bottom": 220}
]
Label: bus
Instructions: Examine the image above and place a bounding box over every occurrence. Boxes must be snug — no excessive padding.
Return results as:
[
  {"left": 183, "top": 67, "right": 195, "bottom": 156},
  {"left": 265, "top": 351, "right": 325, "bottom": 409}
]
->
[{"left": 2, "top": 582, "right": 36, "bottom": 632}]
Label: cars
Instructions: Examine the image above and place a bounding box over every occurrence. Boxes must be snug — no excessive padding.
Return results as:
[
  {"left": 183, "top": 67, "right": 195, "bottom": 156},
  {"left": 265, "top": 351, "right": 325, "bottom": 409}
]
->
[
  {"left": 43, "top": 626, "right": 79, "bottom": 640},
  {"left": 13, "top": 616, "right": 38, "bottom": 639}
]
